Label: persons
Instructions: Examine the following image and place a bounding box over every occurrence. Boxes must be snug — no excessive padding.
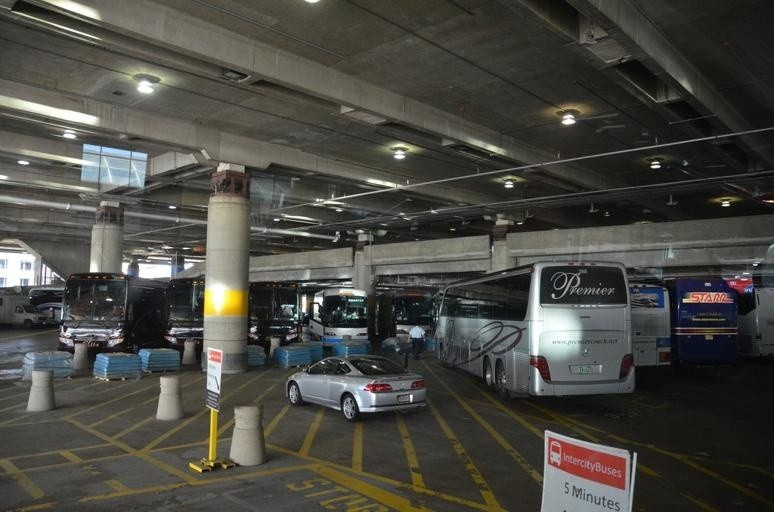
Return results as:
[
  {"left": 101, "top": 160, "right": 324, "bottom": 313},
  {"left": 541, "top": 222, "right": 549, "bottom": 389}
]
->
[{"left": 407, "top": 321, "right": 426, "bottom": 360}]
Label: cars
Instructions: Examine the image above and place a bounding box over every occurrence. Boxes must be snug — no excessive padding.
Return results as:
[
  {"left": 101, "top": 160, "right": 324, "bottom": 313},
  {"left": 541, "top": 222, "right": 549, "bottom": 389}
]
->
[{"left": 285, "top": 354, "right": 428, "bottom": 422}]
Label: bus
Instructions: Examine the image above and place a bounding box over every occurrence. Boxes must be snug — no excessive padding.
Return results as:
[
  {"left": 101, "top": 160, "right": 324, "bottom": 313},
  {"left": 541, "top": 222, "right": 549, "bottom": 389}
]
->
[
  {"left": 248, "top": 282, "right": 302, "bottom": 348},
  {"left": 432, "top": 261, "right": 636, "bottom": 400},
  {"left": 162, "top": 277, "right": 205, "bottom": 352},
  {"left": 56, "top": 272, "right": 169, "bottom": 356}
]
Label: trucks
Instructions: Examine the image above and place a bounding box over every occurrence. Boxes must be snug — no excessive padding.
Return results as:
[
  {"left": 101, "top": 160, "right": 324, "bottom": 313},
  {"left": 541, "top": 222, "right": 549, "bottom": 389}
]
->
[
  {"left": 368, "top": 288, "right": 436, "bottom": 353},
  {"left": 308, "top": 288, "right": 372, "bottom": 349},
  {"left": 0, "top": 286, "right": 49, "bottom": 330}
]
